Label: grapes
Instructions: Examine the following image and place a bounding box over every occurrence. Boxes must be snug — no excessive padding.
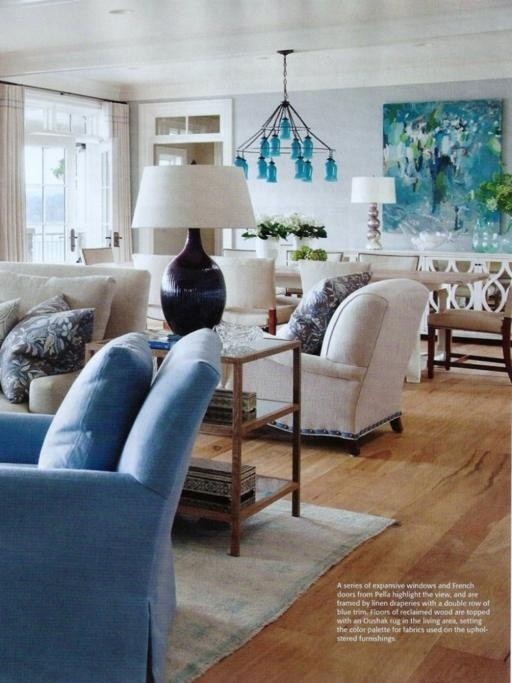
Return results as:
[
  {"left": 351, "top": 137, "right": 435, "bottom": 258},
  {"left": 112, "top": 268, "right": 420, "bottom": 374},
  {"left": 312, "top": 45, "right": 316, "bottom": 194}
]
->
[{"left": 290, "top": 246, "right": 328, "bottom": 261}]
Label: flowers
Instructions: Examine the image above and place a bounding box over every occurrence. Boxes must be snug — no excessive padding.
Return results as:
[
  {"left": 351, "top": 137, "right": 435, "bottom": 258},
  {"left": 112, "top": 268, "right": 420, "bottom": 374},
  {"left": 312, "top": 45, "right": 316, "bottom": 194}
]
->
[
  {"left": 286, "top": 213, "right": 328, "bottom": 240},
  {"left": 241, "top": 214, "right": 288, "bottom": 240}
]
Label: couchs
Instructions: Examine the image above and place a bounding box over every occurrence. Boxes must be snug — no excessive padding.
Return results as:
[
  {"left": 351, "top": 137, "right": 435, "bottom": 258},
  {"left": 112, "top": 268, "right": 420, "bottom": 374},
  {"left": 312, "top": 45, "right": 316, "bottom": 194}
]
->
[
  {"left": 1, "top": 262, "right": 151, "bottom": 412},
  {"left": 0, "top": 327, "right": 224, "bottom": 640},
  {"left": 226, "top": 272, "right": 431, "bottom": 458}
]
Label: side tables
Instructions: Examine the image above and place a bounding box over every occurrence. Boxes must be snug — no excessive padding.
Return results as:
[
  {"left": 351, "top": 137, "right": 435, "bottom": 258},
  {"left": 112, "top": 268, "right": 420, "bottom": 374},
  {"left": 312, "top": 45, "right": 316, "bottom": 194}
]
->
[{"left": 85, "top": 327, "right": 302, "bottom": 556}]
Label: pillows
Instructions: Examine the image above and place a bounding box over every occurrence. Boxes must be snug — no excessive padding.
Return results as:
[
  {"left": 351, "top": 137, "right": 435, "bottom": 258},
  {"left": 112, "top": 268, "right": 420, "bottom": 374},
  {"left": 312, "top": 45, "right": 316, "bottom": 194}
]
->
[
  {"left": 284, "top": 270, "right": 372, "bottom": 353},
  {"left": 1, "top": 297, "right": 19, "bottom": 339},
  {"left": 2, "top": 293, "right": 97, "bottom": 404},
  {"left": 1, "top": 271, "right": 116, "bottom": 341},
  {"left": 38, "top": 333, "right": 153, "bottom": 472}
]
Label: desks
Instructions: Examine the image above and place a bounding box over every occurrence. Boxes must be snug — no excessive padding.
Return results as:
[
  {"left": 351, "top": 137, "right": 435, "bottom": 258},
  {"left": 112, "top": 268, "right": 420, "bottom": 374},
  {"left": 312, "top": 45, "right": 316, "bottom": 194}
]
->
[
  {"left": 273, "top": 264, "right": 304, "bottom": 290},
  {"left": 365, "top": 267, "right": 491, "bottom": 368}
]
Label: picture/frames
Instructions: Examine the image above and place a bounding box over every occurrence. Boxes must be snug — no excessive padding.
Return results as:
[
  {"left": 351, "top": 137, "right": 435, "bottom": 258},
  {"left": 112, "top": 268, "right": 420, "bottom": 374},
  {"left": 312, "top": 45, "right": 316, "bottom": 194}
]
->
[{"left": 380, "top": 98, "right": 511, "bottom": 254}]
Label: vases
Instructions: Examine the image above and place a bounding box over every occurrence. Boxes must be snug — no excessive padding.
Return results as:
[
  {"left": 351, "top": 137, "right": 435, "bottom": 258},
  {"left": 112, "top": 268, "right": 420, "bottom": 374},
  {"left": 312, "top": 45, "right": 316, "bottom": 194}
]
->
[
  {"left": 255, "top": 235, "right": 280, "bottom": 262},
  {"left": 293, "top": 236, "right": 309, "bottom": 253}
]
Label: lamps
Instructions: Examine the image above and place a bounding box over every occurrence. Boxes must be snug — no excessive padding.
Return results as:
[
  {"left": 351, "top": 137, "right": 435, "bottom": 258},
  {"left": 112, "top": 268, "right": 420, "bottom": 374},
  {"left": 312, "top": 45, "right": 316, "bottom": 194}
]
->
[
  {"left": 232, "top": 49, "right": 339, "bottom": 183},
  {"left": 352, "top": 174, "right": 395, "bottom": 253},
  {"left": 129, "top": 165, "right": 256, "bottom": 340}
]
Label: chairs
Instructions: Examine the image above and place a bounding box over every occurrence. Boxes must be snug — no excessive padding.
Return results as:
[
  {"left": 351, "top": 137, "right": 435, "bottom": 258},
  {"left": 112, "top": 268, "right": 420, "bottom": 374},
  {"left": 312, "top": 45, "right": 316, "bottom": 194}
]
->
[
  {"left": 130, "top": 253, "right": 181, "bottom": 308},
  {"left": 426, "top": 269, "right": 511, "bottom": 381},
  {"left": 82, "top": 247, "right": 117, "bottom": 265},
  {"left": 358, "top": 252, "right": 421, "bottom": 281},
  {"left": 206, "top": 255, "right": 296, "bottom": 338},
  {"left": 296, "top": 259, "right": 370, "bottom": 303}
]
